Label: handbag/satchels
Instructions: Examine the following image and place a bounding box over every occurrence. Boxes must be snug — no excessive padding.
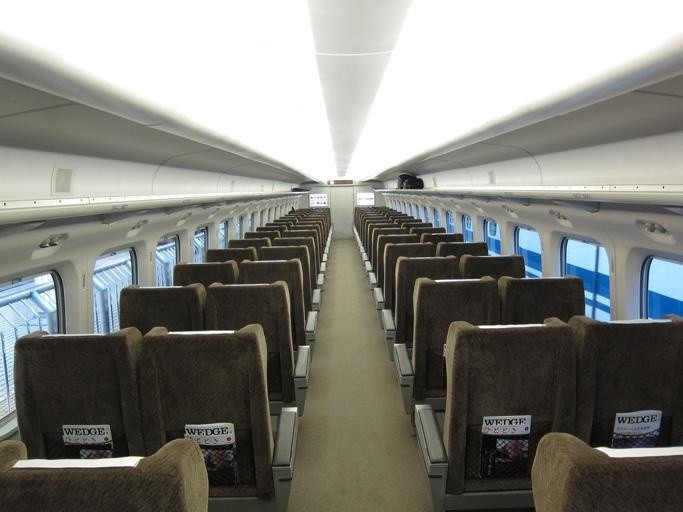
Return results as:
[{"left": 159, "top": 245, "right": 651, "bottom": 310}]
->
[
  {"left": 403, "top": 179, "right": 423, "bottom": 188},
  {"left": 398, "top": 175, "right": 417, "bottom": 189}
]
